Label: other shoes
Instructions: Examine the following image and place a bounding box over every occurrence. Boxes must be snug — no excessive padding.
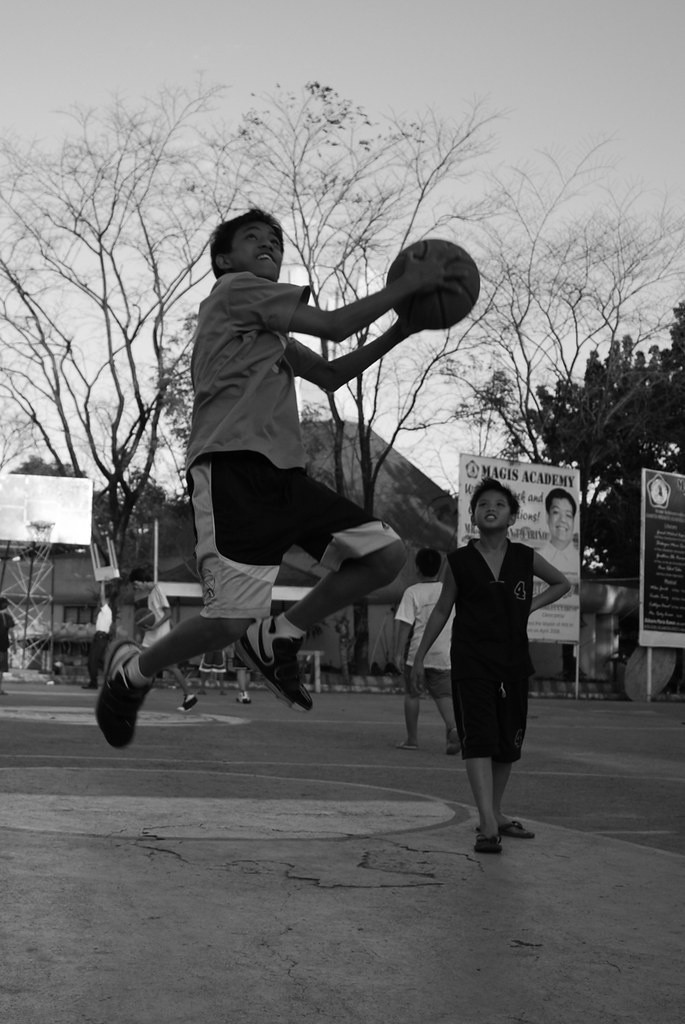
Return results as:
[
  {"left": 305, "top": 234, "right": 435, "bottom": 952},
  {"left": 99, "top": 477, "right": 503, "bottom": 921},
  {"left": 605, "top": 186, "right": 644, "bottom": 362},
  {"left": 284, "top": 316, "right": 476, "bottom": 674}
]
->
[
  {"left": 0, "top": 690, "right": 8, "bottom": 695},
  {"left": 446, "top": 728, "right": 462, "bottom": 754},
  {"left": 237, "top": 698, "right": 251, "bottom": 708},
  {"left": 83, "top": 685, "right": 97, "bottom": 689},
  {"left": 395, "top": 742, "right": 419, "bottom": 750}
]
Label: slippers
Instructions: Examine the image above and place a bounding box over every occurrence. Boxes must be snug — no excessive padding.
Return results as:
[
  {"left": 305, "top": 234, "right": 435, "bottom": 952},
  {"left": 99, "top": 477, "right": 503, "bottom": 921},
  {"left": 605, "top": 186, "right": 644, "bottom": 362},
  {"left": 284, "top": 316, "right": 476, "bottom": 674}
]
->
[
  {"left": 474, "top": 834, "right": 502, "bottom": 853},
  {"left": 476, "top": 817, "right": 534, "bottom": 839}
]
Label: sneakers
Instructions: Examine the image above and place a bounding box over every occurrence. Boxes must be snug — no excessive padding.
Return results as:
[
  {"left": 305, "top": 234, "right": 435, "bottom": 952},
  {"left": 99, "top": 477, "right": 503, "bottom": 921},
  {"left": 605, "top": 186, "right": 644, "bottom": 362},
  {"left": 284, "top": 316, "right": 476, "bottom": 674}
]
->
[
  {"left": 177, "top": 696, "right": 198, "bottom": 712},
  {"left": 236, "top": 616, "right": 313, "bottom": 712},
  {"left": 95, "top": 638, "right": 155, "bottom": 746}
]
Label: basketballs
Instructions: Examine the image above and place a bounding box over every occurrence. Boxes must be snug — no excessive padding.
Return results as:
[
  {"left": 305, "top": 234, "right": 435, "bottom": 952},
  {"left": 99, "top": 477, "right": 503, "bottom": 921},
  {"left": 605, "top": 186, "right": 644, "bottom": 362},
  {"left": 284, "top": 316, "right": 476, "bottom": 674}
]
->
[{"left": 386, "top": 239, "right": 480, "bottom": 329}]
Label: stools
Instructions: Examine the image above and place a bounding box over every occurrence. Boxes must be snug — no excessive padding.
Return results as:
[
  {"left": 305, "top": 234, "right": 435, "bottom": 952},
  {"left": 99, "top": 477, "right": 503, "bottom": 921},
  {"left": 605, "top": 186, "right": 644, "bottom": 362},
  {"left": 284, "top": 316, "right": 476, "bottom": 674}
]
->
[{"left": 90, "top": 537, "right": 120, "bottom": 582}]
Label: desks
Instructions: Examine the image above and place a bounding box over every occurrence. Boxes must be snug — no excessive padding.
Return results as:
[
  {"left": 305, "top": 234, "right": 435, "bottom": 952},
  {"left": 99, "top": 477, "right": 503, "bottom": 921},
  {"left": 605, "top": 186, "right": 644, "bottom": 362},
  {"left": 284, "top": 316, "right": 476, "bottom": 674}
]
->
[{"left": 297, "top": 650, "right": 325, "bottom": 693}]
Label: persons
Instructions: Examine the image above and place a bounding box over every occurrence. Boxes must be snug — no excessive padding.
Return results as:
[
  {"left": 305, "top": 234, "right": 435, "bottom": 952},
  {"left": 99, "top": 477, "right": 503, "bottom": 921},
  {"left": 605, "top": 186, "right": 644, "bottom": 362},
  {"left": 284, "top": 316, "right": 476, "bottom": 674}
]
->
[
  {"left": 130, "top": 568, "right": 198, "bottom": 712},
  {"left": 0, "top": 598, "right": 15, "bottom": 695},
  {"left": 199, "top": 649, "right": 230, "bottom": 695},
  {"left": 233, "top": 642, "right": 251, "bottom": 702},
  {"left": 82, "top": 580, "right": 112, "bottom": 689},
  {"left": 410, "top": 477, "right": 569, "bottom": 852},
  {"left": 95, "top": 208, "right": 468, "bottom": 749},
  {"left": 395, "top": 550, "right": 460, "bottom": 756},
  {"left": 534, "top": 488, "right": 580, "bottom": 583}
]
